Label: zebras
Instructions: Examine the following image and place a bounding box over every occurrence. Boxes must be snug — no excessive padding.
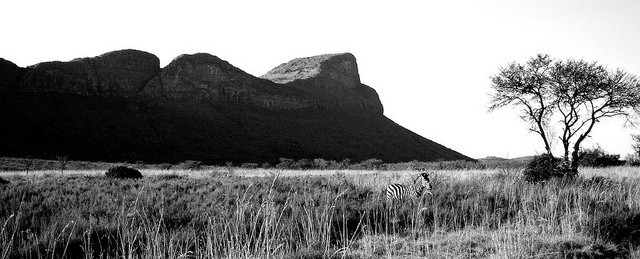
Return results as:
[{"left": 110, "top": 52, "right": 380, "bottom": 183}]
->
[{"left": 385, "top": 172, "right": 432, "bottom": 204}]
